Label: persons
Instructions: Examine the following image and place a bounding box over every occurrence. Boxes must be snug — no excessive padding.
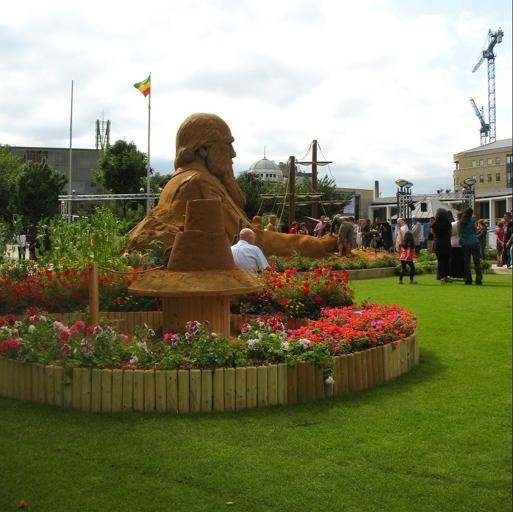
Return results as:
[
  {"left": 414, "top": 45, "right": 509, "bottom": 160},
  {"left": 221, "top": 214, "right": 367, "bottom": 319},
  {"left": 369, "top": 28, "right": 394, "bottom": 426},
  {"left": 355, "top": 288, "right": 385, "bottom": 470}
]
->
[
  {"left": 16, "top": 230, "right": 26, "bottom": 260},
  {"left": 158, "top": 112, "right": 247, "bottom": 228},
  {"left": 229, "top": 228, "right": 274, "bottom": 279},
  {"left": 251, "top": 206, "right": 513, "bottom": 287}
]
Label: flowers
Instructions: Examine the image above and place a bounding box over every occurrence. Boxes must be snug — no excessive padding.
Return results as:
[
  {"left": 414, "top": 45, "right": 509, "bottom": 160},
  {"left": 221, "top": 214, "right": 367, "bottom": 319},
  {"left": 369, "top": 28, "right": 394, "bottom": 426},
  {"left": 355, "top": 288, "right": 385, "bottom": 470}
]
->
[{"left": 1, "top": 257, "right": 418, "bottom": 372}]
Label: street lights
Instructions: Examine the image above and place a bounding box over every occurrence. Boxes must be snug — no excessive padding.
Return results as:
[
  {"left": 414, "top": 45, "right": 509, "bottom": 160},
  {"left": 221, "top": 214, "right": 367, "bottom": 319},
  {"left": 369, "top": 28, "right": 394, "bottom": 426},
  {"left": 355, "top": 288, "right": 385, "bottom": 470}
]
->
[
  {"left": 460, "top": 176, "right": 477, "bottom": 216},
  {"left": 395, "top": 178, "right": 413, "bottom": 229}
]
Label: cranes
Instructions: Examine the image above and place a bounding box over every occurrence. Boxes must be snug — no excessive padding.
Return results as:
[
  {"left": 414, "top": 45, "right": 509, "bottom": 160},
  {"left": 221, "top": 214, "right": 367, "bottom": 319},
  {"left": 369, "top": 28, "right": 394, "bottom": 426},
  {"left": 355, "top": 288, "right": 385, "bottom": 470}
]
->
[{"left": 470, "top": 26, "right": 504, "bottom": 145}]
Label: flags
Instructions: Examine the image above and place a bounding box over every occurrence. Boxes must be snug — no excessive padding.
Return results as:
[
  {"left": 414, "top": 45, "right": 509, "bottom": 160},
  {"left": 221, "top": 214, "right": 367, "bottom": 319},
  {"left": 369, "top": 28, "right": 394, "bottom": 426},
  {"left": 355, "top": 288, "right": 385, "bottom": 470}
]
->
[{"left": 133, "top": 76, "right": 150, "bottom": 98}]
